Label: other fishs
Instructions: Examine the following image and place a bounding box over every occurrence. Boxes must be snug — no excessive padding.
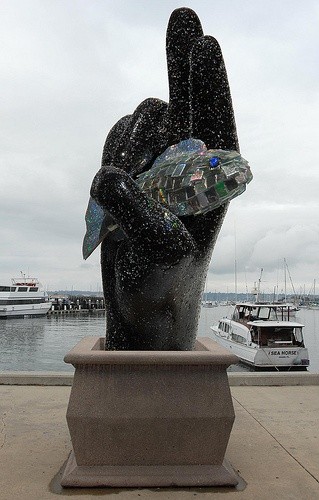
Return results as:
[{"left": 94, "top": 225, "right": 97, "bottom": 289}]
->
[{"left": 81, "top": 138, "right": 253, "bottom": 262}]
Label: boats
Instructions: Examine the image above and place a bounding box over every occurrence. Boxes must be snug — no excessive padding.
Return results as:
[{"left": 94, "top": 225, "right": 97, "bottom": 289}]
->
[
  {"left": 211, "top": 300, "right": 309, "bottom": 370},
  {"left": 0, "top": 273, "right": 52, "bottom": 317}
]
